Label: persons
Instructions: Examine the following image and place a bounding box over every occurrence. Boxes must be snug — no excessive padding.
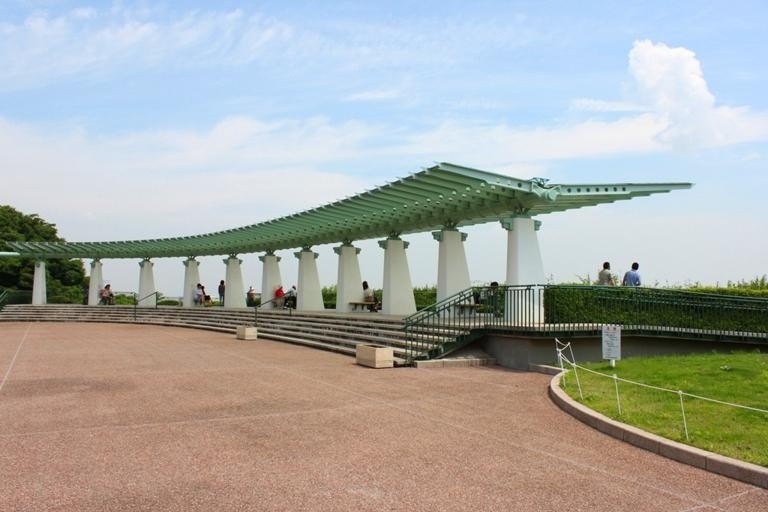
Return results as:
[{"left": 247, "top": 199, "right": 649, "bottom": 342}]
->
[
  {"left": 193, "top": 283, "right": 216, "bottom": 306},
  {"left": 246, "top": 286, "right": 255, "bottom": 307},
  {"left": 218, "top": 280, "right": 225, "bottom": 305},
  {"left": 477, "top": 281, "right": 503, "bottom": 318},
  {"left": 598, "top": 262, "right": 615, "bottom": 287},
  {"left": 285, "top": 285, "right": 297, "bottom": 309},
  {"left": 274, "top": 286, "right": 285, "bottom": 309},
  {"left": 362, "top": 281, "right": 382, "bottom": 313},
  {"left": 83, "top": 284, "right": 115, "bottom": 305},
  {"left": 622, "top": 262, "right": 642, "bottom": 287}
]
